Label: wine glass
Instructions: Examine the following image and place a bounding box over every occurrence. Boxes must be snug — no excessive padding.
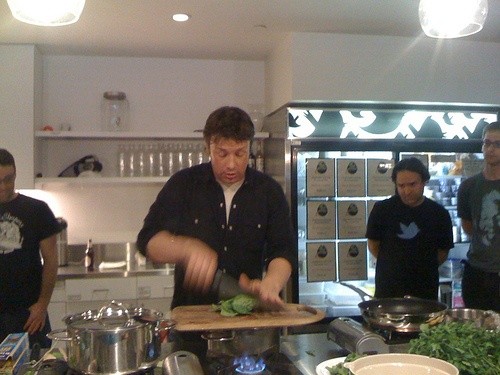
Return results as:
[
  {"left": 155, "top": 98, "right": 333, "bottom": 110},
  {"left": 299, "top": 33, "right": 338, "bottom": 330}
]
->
[{"left": 116, "top": 142, "right": 211, "bottom": 176}]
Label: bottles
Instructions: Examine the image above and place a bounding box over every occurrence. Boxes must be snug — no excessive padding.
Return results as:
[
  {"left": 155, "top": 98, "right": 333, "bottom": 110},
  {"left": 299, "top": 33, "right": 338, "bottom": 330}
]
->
[
  {"left": 247, "top": 141, "right": 265, "bottom": 173},
  {"left": 85, "top": 239, "right": 95, "bottom": 271},
  {"left": 100, "top": 90, "right": 130, "bottom": 133}
]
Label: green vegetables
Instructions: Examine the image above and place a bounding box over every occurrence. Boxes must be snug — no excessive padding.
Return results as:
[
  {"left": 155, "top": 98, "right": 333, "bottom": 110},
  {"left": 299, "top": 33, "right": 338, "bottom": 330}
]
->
[
  {"left": 209, "top": 294, "right": 258, "bottom": 317},
  {"left": 409, "top": 315, "right": 500, "bottom": 375},
  {"left": 324, "top": 352, "right": 368, "bottom": 375}
]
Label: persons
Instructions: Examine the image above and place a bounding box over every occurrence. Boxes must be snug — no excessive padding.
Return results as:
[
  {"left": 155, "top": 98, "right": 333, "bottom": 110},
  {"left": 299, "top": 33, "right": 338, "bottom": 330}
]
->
[
  {"left": 136, "top": 106, "right": 296, "bottom": 358},
  {"left": 0, "top": 148, "right": 62, "bottom": 350},
  {"left": 457, "top": 122, "right": 500, "bottom": 315},
  {"left": 365, "top": 157, "right": 454, "bottom": 301}
]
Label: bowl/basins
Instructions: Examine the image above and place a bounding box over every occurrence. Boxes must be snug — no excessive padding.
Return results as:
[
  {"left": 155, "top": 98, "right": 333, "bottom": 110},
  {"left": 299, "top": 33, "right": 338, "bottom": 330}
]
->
[{"left": 436, "top": 308, "right": 499, "bottom": 332}]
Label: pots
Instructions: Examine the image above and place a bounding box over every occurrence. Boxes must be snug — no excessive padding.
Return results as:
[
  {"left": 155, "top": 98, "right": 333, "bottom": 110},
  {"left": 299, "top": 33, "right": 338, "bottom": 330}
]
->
[
  {"left": 202, "top": 331, "right": 289, "bottom": 360},
  {"left": 335, "top": 279, "right": 446, "bottom": 335},
  {"left": 46, "top": 301, "right": 177, "bottom": 374}
]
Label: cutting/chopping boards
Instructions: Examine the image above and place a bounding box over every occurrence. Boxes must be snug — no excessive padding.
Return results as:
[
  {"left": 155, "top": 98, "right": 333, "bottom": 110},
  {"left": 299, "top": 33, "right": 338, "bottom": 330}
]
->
[{"left": 167, "top": 302, "right": 326, "bottom": 331}]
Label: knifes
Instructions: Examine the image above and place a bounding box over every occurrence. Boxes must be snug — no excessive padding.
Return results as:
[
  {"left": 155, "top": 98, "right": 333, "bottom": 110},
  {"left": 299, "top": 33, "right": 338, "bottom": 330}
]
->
[{"left": 211, "top": 268, "right": 290, "bottom": 313}]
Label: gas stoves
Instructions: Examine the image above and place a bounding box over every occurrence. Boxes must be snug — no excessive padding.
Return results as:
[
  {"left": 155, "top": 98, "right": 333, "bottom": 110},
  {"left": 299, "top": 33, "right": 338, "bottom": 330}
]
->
[
  {"left": 326, "top": 317, "right": 417, "bottom": 355},
  {"left": 202, "top": 352, "right": 303, "bottom": 375}
]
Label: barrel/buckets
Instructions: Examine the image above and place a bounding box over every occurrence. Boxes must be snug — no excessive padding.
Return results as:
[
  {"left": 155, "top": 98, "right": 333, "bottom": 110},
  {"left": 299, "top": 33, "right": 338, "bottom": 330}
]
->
[{"left": 56, "top": 216, "right": 68, "bottom": 266}]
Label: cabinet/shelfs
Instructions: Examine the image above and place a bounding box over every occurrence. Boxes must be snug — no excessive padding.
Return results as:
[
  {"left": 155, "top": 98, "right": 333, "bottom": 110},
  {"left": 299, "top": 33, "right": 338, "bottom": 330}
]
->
[
  {"left": 31, "top": 126, "right": 270, "bottom": 328},
  {"left": 398, "top": 151, "right": 486, "bottom": 242}
]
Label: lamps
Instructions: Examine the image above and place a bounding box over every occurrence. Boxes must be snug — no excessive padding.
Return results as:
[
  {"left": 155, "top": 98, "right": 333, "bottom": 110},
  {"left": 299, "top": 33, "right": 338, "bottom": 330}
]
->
[
  {"left": 7, "top": 0, "right": 85, "bottom": 25},
  {"left": 419, "top": 0, "right": 488, "bottom": 38}
]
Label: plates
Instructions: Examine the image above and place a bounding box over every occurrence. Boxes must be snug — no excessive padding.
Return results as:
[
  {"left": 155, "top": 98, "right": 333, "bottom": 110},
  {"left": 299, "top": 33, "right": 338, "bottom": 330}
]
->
[
  {"left": 316, "top": 356, "right": 348, "bottom": 375},
  {"left": 342, "top": 353, "right": 460, "bottom": 375}
]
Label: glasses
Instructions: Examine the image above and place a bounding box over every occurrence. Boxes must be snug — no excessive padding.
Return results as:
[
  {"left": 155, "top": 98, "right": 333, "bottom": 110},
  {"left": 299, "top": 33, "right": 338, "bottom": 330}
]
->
[{"left": 0, "top": 173, "right": 15, "bottom": 184}]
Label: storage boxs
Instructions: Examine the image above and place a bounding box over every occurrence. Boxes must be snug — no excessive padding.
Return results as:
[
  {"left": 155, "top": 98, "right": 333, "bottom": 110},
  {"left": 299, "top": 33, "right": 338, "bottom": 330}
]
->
[{"left": 0, "top": 332, "right": 30, "bottom": 375}]
